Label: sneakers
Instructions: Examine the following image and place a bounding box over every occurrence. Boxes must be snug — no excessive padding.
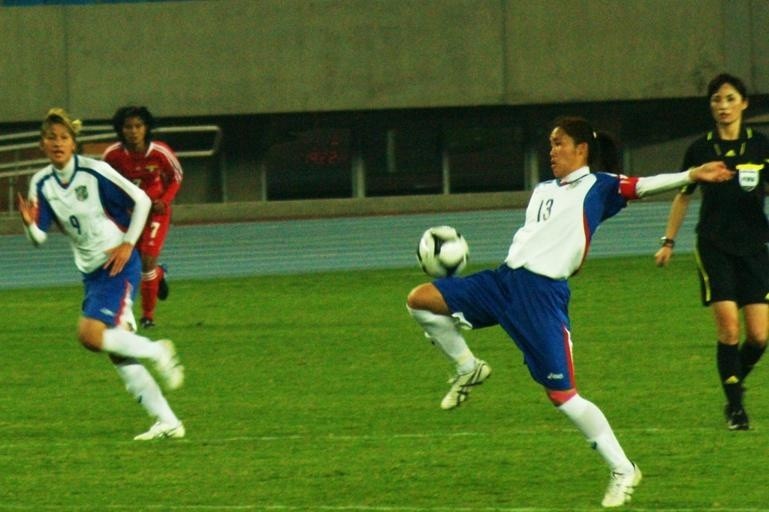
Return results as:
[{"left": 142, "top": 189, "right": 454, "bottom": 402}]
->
[
  {"left": 134, "top": 421, "right": 185, "bottom": 441},
  {"left": 154, "top": 339, "right": 184, "bottom": 390},
  {"left": 158, "top": 262, "right": 168, "bottom": 300},
  {"left": 728, "top": 410, "right": 748, "bottom": 430},
  {"left": 440, "top": 358, "right": 492, "bottom": 411},
  {"left": 601, "top": 461, "right": 642, "bottom": 508},
  {"left": 140, "top": 317, "right": 152, "bottom": 327}
]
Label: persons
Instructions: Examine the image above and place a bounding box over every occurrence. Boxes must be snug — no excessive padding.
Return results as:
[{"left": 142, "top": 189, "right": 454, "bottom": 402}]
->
[
  {"left": 404, "top": 112, "right": 742, "bottom": 509},
  {"left": 650, "top": 72, "right": 767, "bottom": 433},
  {"left": 14, "top": 105, "right": 191, "bottom": 441},
  {"left": 100, "top": 106, "right": 184, "bottom": 332}
]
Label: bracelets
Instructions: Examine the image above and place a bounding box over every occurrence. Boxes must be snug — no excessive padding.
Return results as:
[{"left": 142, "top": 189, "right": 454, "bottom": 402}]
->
[{"left": 660, "top": 236, "right": 674, "bottom": 246}]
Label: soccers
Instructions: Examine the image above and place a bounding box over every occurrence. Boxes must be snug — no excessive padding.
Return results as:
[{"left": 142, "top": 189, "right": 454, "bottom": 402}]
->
[{"left": 416, "top": 225, "right": 470, "bottom": 280}]
